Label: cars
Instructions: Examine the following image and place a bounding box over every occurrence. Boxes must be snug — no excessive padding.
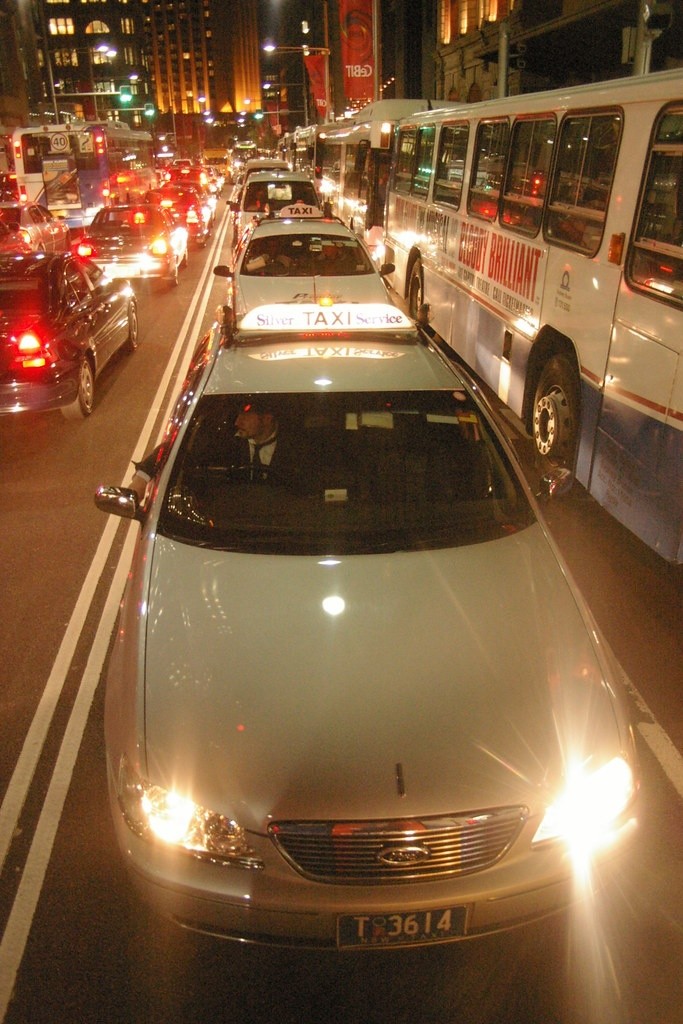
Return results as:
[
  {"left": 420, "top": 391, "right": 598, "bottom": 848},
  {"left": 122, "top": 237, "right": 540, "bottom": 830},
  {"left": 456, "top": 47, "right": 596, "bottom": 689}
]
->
[
  {"left": 75, "top": 205, "right": 191, "bottom": 292},
  {"left": 213, "top": 214, "right": 401, "bottom": 347},
  {"left": 1, "top": 200, "right": 72, "bottom": 258},
  {"left": 237, "top": 158, "right": 291, "bottom": 187},
  {"left": 172, "top": 141, "right": 274, "bottom": 194},
  {"left": 145, "top": 188, "right": 212, "bottom": 245},
  {"left": 226, "top": 169, "right": 334, "bottom": 251},
  {"left": 91, "top": 340, "right": 643, "bottom": 956}
]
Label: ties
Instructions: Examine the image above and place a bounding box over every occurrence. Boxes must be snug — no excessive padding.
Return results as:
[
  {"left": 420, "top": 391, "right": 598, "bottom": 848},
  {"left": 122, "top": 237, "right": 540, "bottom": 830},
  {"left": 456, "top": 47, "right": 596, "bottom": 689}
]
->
[{"left": 251, "top": 437, "right": 277, "bottom": 483}]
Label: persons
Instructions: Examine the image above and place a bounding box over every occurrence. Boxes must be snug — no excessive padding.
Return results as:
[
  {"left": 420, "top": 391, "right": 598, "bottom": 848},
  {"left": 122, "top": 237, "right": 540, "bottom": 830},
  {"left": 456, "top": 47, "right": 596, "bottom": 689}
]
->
[
  {"left": 128, "top": 403, "right": 297, "bottom": 503},
  {"left": 247, "top": 191, "right": 269, "bottom": 212},
  {"left": 322, "top": 241, "right": 337, "bottom": 261}
]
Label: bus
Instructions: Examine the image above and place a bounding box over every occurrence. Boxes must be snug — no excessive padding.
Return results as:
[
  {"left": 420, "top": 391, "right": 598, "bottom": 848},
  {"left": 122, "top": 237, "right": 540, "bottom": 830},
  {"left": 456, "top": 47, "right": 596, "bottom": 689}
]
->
[
  {"left": 380, "top": 67, "right": 682, "bottom": 572},
  {"left": 10, "top": 120, "right": 158, "bottom": 229},
  {"left": 320, "top": 97, "right": 467, "bottom": 249},
  {"left": 276, "top": 123, "right": 341, "bottom": 175}
]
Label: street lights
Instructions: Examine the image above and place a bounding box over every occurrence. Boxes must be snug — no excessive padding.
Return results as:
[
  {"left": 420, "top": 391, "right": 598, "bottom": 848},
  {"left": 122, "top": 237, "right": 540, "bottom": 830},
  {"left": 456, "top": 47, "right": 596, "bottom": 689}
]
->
[
  {"left": 264, "top": 43, "right": 332, "bottom": 125},
  {"left": 261, "top": 83, "right": 310, "bottom": 129},
  {"left": 45, "top": 42, "right": 118, "bottom": 126},
  {"left": 91, "top": 75, "right": 144, "bottom": 120}
]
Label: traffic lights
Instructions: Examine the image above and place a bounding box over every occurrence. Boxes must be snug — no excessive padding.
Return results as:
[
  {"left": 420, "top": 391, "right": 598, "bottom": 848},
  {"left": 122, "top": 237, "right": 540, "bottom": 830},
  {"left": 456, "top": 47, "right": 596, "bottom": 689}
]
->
[
  {"left": 145, "top": 104, "right": 158, "bottom": 123},
  {"left": 120, "top": 86, "right": 133, "bottom": 108}
]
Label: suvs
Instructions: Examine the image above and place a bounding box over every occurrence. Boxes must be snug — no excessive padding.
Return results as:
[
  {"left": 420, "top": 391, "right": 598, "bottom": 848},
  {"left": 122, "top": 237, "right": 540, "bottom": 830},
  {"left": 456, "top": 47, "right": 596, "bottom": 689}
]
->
[{"left": 0, "top": 252, "right": 136, "bottom": 421}]
